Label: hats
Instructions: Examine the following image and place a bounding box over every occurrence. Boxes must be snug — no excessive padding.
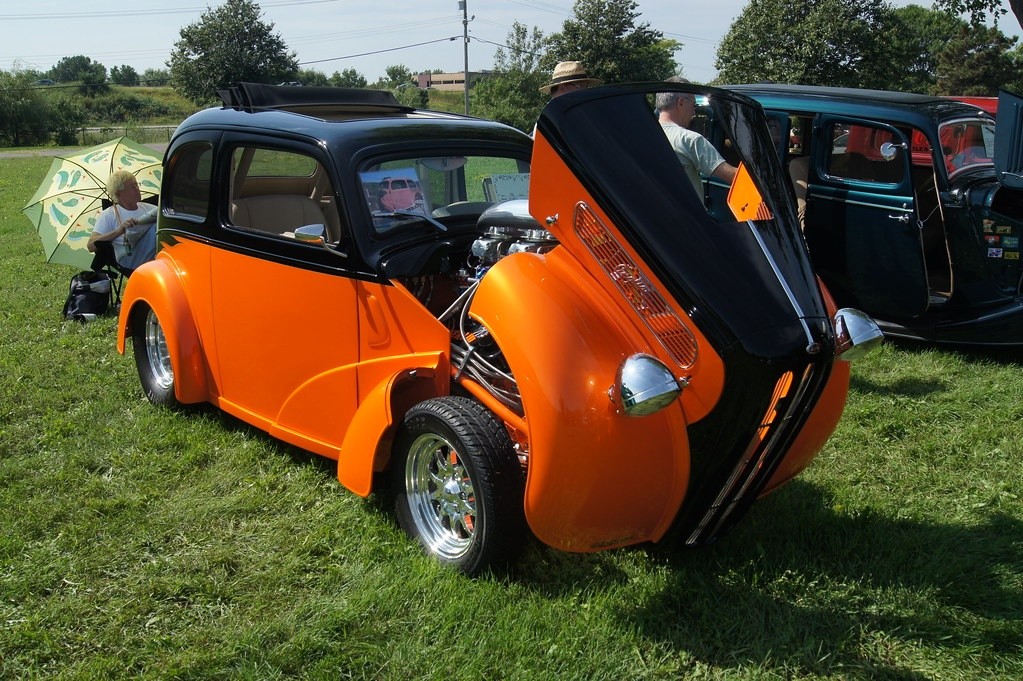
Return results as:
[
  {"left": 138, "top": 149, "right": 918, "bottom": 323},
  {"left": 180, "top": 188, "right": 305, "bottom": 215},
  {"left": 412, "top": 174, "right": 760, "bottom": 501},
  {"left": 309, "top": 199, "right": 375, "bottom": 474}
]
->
[{"left": 539, "top": 60, "right": 603, "bottom": 94}]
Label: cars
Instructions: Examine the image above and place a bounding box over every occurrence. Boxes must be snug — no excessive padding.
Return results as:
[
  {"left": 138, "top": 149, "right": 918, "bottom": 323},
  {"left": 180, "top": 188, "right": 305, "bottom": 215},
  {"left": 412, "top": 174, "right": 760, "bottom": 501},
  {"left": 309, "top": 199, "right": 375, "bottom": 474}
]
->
[
  {"left": 702, "top": 78, "right": 1023, "bottom": 368},
  {"left": 113, "top": 79, "right": 889, "bottom": 584}
]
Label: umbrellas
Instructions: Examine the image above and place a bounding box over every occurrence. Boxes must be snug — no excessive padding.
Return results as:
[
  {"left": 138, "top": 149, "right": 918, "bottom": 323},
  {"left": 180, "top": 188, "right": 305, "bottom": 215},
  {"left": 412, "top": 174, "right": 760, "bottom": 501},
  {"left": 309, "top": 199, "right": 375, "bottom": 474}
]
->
[{"left": 16, "top": 135, "right": 162, "bottom": 270}]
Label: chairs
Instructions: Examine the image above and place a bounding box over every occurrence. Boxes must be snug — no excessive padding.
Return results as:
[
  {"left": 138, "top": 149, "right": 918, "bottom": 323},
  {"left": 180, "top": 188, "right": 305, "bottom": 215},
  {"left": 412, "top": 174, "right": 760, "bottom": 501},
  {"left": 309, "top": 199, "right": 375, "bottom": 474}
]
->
[
  {"left": 230, "top": 175, "right": 334, "bottom": 245},
  {"left": 92, "top": 194, "right": 159, "bottom": 309},
  {"left": 791, "top": 156, "right": 811, "bottom": 235}
]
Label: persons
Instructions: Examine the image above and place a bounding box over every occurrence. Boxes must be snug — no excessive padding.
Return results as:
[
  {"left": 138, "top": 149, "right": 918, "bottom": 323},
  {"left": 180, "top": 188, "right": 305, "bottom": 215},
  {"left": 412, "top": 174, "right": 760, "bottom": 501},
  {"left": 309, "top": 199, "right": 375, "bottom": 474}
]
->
[
  {"left": 529, "top": 60, "right": 600, "bottom": 138},
  {"left": 82, "top": 170, "right": 162, "bottom": 268},
  {"left": 650, "top": 75, "right": 747, "bottom": 214}
]
